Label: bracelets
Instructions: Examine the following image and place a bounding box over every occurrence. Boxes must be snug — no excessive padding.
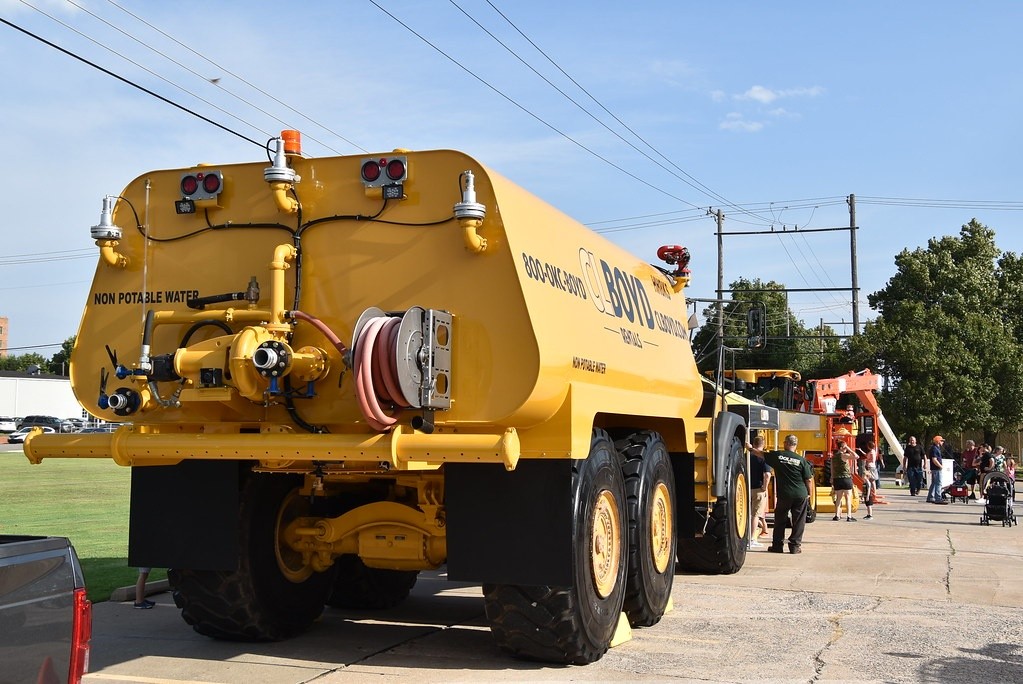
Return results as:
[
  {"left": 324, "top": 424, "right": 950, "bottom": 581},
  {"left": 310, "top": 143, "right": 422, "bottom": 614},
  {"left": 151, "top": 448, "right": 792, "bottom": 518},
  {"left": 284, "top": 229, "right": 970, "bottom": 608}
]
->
[{"left": 749, "top": 448, "right": 753, "bottom": 452}]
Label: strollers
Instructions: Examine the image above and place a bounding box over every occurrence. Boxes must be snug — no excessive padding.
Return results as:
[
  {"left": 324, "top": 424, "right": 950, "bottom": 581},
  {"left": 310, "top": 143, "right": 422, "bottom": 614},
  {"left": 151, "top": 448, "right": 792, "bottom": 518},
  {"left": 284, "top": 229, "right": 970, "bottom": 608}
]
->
[
  {"left": 979, "top": 471, "right": 1018, "bottom": 527},
  {"left": 941, "top": 469, "right": 976, "bottom": 500}
]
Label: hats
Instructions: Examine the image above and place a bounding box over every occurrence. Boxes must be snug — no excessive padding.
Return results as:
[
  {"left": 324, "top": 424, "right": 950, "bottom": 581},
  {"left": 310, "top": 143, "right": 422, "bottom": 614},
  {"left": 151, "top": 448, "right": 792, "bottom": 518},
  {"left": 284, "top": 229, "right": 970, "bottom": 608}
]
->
[{"left": 934, "top": 436, "right": 945, "bottom": 443}]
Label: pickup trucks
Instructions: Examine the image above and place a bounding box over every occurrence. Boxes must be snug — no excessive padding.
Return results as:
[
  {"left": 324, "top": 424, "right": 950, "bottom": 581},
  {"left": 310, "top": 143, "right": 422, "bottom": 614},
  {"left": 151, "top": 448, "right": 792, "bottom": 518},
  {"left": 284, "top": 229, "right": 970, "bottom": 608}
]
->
[{"left": 1, "top": 533, "right": 92, "bottom": 683}]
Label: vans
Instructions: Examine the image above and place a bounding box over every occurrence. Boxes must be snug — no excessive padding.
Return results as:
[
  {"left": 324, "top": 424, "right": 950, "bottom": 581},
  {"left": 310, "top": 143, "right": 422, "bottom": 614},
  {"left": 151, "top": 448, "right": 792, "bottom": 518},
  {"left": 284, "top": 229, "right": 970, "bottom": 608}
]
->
[{"left": 23, "top": 416, "right": 76, "bottom": 434}]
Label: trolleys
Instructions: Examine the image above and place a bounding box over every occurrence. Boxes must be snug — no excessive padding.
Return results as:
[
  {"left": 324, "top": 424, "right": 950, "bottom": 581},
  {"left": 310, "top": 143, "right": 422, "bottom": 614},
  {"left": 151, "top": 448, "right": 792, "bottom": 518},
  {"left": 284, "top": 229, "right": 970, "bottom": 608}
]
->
[{"left": 949, "top": 484, "right": 969, "bottom": 504}]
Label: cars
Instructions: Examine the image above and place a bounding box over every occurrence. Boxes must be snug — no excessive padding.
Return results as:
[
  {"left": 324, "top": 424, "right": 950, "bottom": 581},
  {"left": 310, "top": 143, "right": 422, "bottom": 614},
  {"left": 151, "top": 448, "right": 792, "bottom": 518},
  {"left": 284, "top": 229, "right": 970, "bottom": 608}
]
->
[
  {"left": 12, "top": 417, "right": 24, "bottom": 431},
  {"left": 0, "top": 416, "right": 16, "bottom": 435},
  {"left": 66, "top": 418, "right": 131, "bottom": 434},
  {"left": 7, "top": 426, "right": 55, "bottom": 444}
]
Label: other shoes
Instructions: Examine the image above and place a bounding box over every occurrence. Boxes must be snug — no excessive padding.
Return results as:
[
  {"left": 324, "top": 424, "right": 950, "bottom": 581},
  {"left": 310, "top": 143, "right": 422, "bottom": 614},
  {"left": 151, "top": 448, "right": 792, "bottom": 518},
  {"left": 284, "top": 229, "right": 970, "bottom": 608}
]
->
[
  {"left": 144, "top": 600, "right": 155, "bottom": 605},
  {"left": 759, "top": 532, "right": 769, "bottom": 538},
  {"left": 133, "top": 602, "right": 152, "bottom": 609},
  {"left": 768, "top": 546, "right": 784, "bottom": 553},
  {"left": 927, "top": 499, "right": 935, "bottom": 502},
  {"left": 935, "top": 500, "right": 948, "bottom": 504},
  {"left": 833, "top": 516, "right": 839, "bottom": 521},
  {"left": 847, "top": 517, "right": 857, "bottom": 522},
  {"left": 970, "top": 492, "right": 976, "bottom": 498},
  {"left": 790, "top": 548, "right": 801, "bottom": 554},
  {"left": 864, "top": 514, "right": 873, "bottom": 520},
  {"left": 916, "top": 489, "right": 920, "bottom": 494},
  {"left": 911, "top": 492, "right": 916, "bottom": 496}
]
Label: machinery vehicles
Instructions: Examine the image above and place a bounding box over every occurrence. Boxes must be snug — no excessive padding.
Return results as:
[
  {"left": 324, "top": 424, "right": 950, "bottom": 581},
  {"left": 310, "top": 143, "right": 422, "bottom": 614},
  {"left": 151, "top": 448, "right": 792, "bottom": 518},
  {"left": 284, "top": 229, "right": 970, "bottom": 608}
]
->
[
  {"left": 706, "top": 365, "right": 911, "bottom": 515},
  {"left": 25, "top": 129, "right": 776, "bottom": 668}
]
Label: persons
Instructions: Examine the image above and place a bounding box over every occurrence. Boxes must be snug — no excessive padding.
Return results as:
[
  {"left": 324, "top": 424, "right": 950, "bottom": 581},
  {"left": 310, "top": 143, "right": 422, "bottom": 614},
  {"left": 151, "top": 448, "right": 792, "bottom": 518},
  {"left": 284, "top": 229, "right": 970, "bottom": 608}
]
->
[
  {"left": 1004, "top": 458, "right": 1016, "bottom": 502},
  {"left": 876, "top": 446, "right": 885, "bottom": 491},
  {"left": 861, "top": 473, "right": 874, "bottom": 520},
  {"left": 926, "top": 436, "right": 948, "bottom": 505},
  {"left": 993, "top": 445, "right": 1012, "bottom": 477},
  {"left": 745, "top": 434, "right": 814, "bottom": 553},
  {"left": 857, "top": 441, "right": 879, "bottom": 501},
  {"left": 903, "top": 436, "right": 927, "bottom": 496},
  {"left": 747, "top": 436, "right": 772, "bottom": 548},
  {"left": 829, "top": 438, "right": 860, "bottom": 522},
  {"left": 955, "top": 439, "right": 979, "bottom": 500},
  {"left": 971, "top": 442, "right": 1002, "bottom": 503}
]
